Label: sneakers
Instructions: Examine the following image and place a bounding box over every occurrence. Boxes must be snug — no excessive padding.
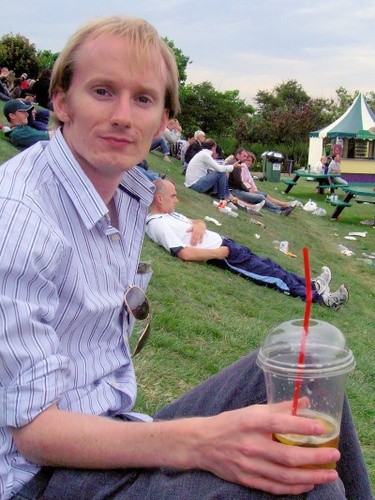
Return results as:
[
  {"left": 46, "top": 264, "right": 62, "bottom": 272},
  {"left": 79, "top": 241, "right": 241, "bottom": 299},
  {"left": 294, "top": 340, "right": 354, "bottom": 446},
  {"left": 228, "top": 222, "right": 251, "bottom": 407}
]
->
[
  {"left": 313, "top": 266, "right": 331, "bottom": 295},
  {"left": 325, "top": 284, "right": 349, "bottom": 311}
]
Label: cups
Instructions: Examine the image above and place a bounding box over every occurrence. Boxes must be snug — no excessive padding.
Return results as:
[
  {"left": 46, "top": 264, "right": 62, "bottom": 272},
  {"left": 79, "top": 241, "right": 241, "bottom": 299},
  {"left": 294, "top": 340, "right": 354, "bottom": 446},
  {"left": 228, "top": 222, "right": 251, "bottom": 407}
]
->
[{"left": 254, "top": 318, "right": 357, "bottom": 472}]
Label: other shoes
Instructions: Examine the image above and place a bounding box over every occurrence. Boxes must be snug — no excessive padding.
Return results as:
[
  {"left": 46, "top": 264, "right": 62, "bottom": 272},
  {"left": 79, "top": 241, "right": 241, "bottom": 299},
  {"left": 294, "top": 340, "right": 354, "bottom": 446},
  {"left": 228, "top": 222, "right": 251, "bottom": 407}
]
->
[
  {"left": 288, "top": 200, "right": 298, "bottom": 209},
  {"left": 226, "top": 201, "right": 238, "bottom": 211},
  {"left": 255, "top": 200, "right": 265, "bottom": 212}
]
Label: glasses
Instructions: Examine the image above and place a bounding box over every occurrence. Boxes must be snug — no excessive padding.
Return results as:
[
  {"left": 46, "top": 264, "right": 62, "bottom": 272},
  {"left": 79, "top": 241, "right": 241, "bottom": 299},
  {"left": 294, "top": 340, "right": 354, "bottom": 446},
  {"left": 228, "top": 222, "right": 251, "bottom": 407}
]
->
[{"left": 124, "top": 287, "right": 151, "bottom": 358}]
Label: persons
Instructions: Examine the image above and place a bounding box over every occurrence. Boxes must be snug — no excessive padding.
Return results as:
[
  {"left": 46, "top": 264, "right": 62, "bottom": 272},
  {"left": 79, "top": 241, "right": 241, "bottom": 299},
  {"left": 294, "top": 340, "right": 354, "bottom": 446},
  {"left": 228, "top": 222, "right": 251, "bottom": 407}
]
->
[
  {"left": 149, "top": 118, "right": 298, "bottom": 216},
  {"left": 315, "top": 154, "right": 356, "bottom": 198},
  {"left": 0, "top": 16, "right": 375, "bottom": 500},
  {"left": 145, "top": 180, "right": 351, "bottom": 311},
  {"left": 0, "top": 67, "right": 58, "bottom": 150}
]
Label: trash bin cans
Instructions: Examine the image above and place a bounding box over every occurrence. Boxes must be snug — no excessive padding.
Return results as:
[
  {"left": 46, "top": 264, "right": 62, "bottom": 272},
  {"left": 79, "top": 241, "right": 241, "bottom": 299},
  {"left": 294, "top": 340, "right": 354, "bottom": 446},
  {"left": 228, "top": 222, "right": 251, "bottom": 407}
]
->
[{"left": 262, "top": 152, "right": 283, "bottom": 182}]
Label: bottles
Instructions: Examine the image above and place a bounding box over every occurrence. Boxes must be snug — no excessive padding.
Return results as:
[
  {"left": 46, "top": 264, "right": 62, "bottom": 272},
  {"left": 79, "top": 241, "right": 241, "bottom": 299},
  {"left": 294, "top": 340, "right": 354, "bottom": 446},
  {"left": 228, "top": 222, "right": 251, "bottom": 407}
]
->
[{"left": 0, "top": 125, "right": 11, "bottom": 132}]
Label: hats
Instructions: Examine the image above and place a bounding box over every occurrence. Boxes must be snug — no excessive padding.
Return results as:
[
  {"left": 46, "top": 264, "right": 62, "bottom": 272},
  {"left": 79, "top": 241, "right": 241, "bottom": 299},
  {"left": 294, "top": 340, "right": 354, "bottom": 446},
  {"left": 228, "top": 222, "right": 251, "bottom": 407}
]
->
[{"left": 4, "top": 99, "right": 34, "bottom": 115}]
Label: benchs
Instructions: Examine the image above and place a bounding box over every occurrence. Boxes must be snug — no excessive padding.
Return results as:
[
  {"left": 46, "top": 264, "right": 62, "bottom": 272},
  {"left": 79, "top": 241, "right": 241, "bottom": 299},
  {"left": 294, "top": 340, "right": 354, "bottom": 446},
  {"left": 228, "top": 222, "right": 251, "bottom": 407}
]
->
[{"left": 285, "top": 179, "right": 375, "bottom": 205}]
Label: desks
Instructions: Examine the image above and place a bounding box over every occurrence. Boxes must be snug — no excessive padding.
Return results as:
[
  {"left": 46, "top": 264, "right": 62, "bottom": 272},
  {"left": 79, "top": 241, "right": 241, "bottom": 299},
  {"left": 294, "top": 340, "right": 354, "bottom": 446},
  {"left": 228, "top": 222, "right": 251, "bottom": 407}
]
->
[
  {"left": 284, "top": 170, "right": 342, "bottom": 194},
  {"left": 331, "top": 187, "right": 375, "bottom": 219}
]
